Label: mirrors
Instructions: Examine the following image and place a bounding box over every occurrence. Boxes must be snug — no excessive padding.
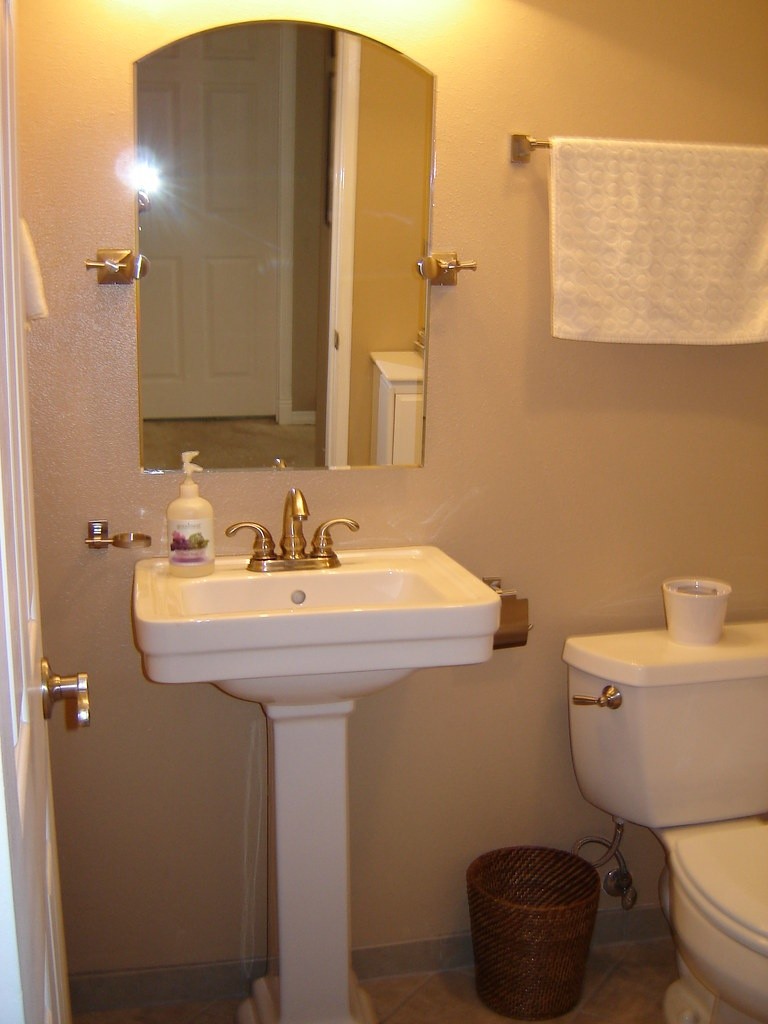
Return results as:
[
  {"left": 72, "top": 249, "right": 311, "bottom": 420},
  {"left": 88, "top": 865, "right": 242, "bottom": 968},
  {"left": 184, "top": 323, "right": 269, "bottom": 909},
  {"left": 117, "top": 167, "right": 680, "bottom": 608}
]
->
[{"left": 131, "top": 17, "right": 436, "bottom": 476}]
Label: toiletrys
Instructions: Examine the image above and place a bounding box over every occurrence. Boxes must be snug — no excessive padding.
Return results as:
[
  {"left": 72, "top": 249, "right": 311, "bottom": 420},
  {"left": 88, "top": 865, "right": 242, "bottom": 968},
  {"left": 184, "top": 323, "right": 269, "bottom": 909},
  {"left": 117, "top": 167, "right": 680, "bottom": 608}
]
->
[
  {"left": 166, "top": 463, "right": 216, "bottom": 578},
  {"left": 181, "top": 448, "right": 200, "bottom": 468}
]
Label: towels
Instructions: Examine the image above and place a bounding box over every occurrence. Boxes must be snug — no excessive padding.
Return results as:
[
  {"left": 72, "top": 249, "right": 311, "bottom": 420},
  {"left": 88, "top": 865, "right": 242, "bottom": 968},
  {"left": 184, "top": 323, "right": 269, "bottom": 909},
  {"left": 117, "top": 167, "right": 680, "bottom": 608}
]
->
[
  {"left": 547, "top": 137, "right": 768, "bottom": 348},
  {"left": 20, "top": 218, "right": 50, "bottom": 321}
]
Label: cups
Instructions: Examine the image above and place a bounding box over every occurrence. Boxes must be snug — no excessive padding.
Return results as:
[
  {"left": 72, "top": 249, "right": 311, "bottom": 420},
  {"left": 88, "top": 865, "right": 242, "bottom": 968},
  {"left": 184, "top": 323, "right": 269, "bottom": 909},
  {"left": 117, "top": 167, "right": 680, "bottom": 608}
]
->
[{"left": 663, "top": 578, "right": 732, "bottom": 646}]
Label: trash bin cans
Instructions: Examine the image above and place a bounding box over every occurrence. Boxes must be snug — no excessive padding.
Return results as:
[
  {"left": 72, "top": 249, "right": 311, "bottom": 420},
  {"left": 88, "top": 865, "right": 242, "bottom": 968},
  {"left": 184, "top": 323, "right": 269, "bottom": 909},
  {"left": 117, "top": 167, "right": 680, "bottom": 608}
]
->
[{"left": 466, "top": 845, "right": 602, "bottom": 1021}]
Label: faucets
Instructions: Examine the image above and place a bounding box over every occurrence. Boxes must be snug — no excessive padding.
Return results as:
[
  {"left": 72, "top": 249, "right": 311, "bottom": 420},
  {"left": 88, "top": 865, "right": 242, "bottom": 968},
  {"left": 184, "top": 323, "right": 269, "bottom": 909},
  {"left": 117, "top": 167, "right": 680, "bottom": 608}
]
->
[
  {"left": 278, "top": 487, "right": 314, "bottom": 570},
  {"left": 271, "top": 457, "right": 288, "bottom": 468}
]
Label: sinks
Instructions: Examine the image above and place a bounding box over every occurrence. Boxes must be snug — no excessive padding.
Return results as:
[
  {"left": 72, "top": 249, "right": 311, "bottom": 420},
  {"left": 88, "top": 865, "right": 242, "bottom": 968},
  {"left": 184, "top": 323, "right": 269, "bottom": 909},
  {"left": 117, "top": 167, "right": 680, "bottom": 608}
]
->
[{"left": 132, "top": 545, "right": 502, "bottom": 685}]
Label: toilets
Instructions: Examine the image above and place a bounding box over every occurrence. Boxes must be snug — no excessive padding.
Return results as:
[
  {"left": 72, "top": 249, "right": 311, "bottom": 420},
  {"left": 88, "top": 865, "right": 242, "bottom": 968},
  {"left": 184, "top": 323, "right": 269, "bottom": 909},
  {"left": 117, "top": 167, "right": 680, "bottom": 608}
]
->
[{"left": 561, "top": 621, "right": 768, "bottom": 1024}]
